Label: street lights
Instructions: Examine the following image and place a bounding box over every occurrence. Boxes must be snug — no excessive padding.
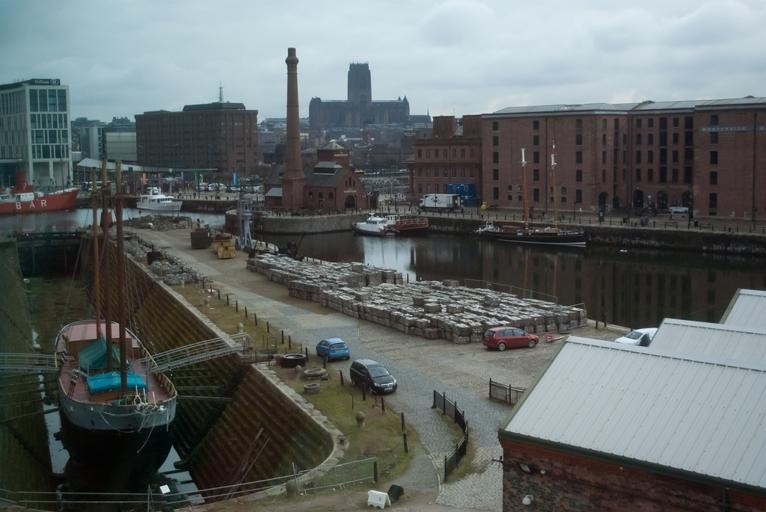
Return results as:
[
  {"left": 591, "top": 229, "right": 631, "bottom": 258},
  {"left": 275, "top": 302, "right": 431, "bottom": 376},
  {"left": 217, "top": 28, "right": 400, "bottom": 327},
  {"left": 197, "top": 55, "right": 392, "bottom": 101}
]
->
[{"left": 385, "top": 179, "right": 400, "bottom": 199}]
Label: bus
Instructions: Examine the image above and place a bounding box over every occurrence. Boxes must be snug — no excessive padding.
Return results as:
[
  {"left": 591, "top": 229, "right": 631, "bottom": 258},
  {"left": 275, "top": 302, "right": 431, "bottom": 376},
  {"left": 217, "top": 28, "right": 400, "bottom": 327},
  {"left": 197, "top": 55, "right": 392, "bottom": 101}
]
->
[{"left": 87, "top": 181, "right": 111, "bottom": 191}]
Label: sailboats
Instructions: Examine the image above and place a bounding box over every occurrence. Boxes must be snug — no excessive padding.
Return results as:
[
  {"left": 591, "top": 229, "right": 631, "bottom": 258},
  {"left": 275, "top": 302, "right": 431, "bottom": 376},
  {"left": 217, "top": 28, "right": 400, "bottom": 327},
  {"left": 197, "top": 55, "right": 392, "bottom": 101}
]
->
[
  {"left": 472, "top": 147, "right": 588, "bottom": 248},
  {"left": 53, "top": 156, "right": 178, "bottom": 433}
]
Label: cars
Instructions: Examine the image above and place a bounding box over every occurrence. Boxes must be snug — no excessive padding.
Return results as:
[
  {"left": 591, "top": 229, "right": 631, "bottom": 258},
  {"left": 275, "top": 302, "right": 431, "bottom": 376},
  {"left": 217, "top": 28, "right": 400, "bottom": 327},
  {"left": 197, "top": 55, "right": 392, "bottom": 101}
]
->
[
  {"left": 483, "top": 326, "right": 539, "bottom": 352},
  {"left": 194, "top": 182, "right": 264, "bottom": 194},
  {"left": 668, "top": 204, "right": 689, "bottom": 213},
  {"left": 615, "top": 327, "right": 659, "bottom": 346},
  {"left": 316, "top": 337, "right": 350, "bottom": 362}
]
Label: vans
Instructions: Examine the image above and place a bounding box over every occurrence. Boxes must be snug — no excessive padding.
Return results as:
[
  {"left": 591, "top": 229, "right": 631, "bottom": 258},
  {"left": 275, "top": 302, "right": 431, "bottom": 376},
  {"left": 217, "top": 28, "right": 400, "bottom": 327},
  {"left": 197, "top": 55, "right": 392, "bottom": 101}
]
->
[{"left": 350, "top": 358, "right": 398, "bottom": 395}]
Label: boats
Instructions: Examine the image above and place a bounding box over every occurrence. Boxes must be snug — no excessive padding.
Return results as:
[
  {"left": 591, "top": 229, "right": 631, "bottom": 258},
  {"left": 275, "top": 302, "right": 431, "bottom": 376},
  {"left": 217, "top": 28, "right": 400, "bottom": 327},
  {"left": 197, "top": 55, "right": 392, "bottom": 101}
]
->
[
  {"left": 136, "top": 186, "right": 183, "bottom": 212},
  {"left": 0, "top": 171, "right": 80, "bottom": 215},
  {"left": 352, "top": 211, "right": 429, "bottom": 237}
]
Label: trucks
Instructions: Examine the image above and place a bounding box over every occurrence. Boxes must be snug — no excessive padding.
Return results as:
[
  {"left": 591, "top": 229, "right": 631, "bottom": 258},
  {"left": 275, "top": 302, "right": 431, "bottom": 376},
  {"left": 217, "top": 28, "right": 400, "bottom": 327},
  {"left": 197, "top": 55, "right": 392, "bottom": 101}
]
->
[{"left": 419, "top": 193, "right": 461, "bottom": 212}]
